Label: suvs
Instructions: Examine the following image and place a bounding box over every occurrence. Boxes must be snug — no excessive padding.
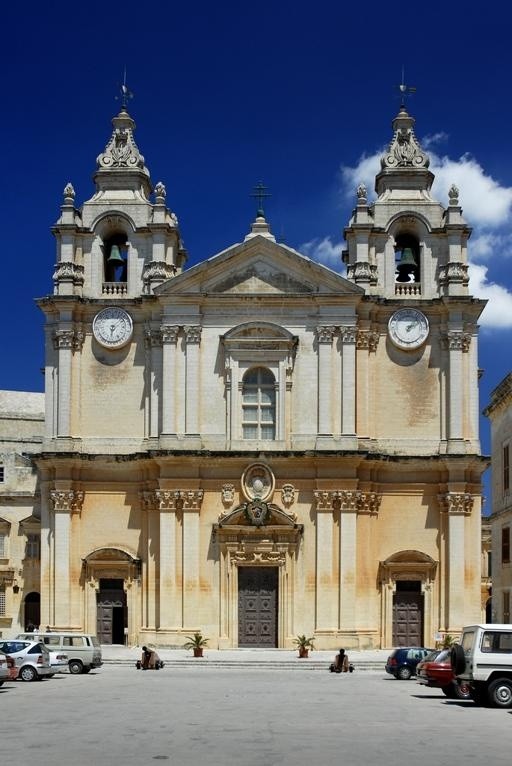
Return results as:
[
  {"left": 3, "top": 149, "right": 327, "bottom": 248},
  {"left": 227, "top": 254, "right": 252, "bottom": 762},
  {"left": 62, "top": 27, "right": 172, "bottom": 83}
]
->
[{"left": 451, "top": 624, "right": 512, "bottom": 711}]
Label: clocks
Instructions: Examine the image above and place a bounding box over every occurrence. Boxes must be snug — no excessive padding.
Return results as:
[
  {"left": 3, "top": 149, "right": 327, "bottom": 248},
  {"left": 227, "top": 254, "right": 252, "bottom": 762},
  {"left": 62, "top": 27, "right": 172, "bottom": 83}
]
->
[
  {"left": 93, "top": 307, "right": 133, "bottom": 350},
  {"left": 387, "top": 308, "right": 429, "bottom": 351}
]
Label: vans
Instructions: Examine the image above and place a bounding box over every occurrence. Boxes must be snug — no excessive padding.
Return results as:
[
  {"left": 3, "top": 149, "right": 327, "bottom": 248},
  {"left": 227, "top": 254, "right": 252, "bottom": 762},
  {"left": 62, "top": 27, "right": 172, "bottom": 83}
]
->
[
  {"left": 16, "top": 633, "right": 103, "bottom": 676},
  {"left": 0, "top": 640, "right": 53, "bottom": 681}
]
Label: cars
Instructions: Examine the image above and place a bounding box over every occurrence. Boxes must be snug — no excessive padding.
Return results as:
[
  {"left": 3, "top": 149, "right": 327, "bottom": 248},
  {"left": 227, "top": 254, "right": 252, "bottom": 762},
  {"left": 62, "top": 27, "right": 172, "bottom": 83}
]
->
[
  {"left": 385, "top": 647, "right": 433, "bottom": 683},
  {"left": 0, "top": 651, "right": 18, "bottom": 688},
  {"left": 45, "top": 648, "right": 69, "bottom": 680},
  {"left": 417, "top": 647, "right": 469, "bottom": 701}
]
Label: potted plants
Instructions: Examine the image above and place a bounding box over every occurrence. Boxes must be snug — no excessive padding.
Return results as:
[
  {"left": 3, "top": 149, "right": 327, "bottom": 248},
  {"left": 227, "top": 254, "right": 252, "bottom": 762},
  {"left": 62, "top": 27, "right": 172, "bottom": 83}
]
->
[
  {"left": 184, "top": 633, "right": 208, "bottom": 656},
  {"left": 292, "top": 634, "right": 315, "bottom": 657}
]
